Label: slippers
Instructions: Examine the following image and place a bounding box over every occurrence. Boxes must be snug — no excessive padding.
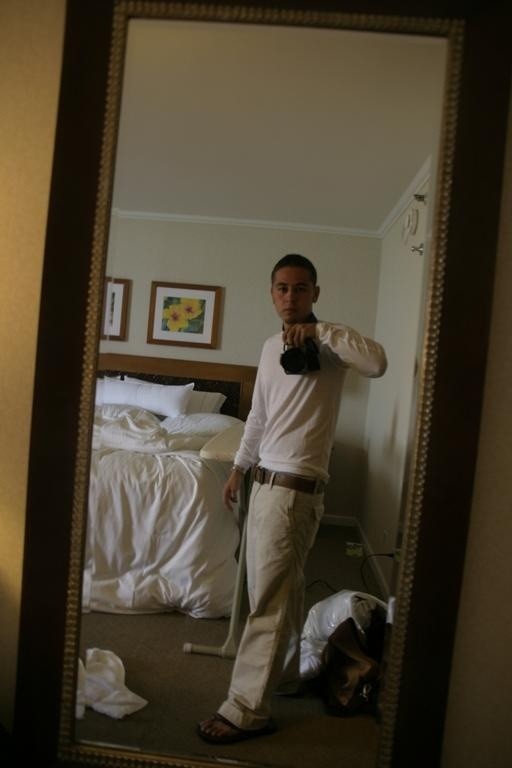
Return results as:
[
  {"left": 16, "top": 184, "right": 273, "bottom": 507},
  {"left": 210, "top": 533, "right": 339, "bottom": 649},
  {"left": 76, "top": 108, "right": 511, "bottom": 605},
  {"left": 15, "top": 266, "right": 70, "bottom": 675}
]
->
[{"left": 195, "top": 713, "right": 275, "bottom": 745}]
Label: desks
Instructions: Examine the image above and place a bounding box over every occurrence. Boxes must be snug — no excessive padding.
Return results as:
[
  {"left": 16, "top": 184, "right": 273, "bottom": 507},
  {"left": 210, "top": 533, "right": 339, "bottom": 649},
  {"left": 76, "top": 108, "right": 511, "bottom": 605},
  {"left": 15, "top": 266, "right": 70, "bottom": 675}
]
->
[{"left": 183, "top": 421, "right": 248, "bottom": 660}]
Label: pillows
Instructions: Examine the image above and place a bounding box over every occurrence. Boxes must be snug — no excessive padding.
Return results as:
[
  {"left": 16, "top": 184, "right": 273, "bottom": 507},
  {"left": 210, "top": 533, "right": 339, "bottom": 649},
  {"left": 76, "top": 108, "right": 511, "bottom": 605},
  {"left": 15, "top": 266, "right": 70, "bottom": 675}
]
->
[
  {"left": 102, "top": 375, "right": 195, "bottom": 419},
  {"left": 158, "top": 413, "right": 243, "bottom": 435},
  {"left": 124, "top": 375, "right": 227, "bottom": 413},
  {"left": 95, "top": 375, "right": 122, "bottom": 406}
]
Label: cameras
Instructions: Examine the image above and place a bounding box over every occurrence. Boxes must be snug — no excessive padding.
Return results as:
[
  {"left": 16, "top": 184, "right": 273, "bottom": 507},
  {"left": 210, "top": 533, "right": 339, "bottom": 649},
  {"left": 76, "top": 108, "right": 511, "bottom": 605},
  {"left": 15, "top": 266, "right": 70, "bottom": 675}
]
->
[{"left": 279, "top": 338, "right": 320, "bottom": 376}]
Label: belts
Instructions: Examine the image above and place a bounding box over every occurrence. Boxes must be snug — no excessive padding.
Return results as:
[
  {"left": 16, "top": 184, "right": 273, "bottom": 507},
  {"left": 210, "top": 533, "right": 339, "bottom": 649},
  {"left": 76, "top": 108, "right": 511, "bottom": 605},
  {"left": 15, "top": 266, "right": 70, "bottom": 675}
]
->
[{"left": 254, "top": 466, "right": 324, "bottom": 495}]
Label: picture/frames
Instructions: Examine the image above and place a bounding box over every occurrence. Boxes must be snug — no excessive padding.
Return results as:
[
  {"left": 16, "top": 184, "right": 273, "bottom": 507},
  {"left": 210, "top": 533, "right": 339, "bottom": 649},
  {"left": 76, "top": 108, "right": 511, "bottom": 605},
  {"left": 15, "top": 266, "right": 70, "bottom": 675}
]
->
[
  {"left": 146, "top": 281, "right": 222, "bottom": 349},
  {"left": 11, "top": 1, "right": 512, "bottom": 768},
  {"left": 100, "top": 277, "right": 129, "bottom": 341}
]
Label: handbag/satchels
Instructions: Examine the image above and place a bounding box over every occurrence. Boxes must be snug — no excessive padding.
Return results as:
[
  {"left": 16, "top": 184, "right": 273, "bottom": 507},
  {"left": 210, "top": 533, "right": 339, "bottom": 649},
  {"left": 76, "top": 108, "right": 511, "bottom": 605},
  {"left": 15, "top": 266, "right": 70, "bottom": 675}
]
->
[{"left": 300, "top": 588, "right": 394, "bottom": 717}]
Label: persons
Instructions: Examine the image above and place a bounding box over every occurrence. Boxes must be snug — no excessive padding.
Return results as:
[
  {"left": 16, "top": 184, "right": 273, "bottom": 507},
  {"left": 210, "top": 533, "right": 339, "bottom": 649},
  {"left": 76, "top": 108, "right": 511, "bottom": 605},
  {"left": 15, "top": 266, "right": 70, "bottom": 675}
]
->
[{"left": 192, "top": 253, "right": 386, "bottom": 745}]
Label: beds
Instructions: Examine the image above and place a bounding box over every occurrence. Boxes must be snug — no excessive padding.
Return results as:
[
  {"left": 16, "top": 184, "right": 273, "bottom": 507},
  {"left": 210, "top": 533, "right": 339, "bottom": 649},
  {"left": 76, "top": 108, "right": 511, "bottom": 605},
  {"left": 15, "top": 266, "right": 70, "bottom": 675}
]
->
[{"left": 82, "top": 354, "right": 258, "bottom": 618}]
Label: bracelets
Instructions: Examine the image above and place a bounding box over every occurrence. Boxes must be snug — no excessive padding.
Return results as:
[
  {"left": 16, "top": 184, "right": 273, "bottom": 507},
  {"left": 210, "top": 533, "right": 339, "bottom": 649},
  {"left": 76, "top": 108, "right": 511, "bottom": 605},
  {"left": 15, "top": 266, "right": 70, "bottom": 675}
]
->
[{"left": 229, "top": 465, "right": 246, "bottom": 476}]
